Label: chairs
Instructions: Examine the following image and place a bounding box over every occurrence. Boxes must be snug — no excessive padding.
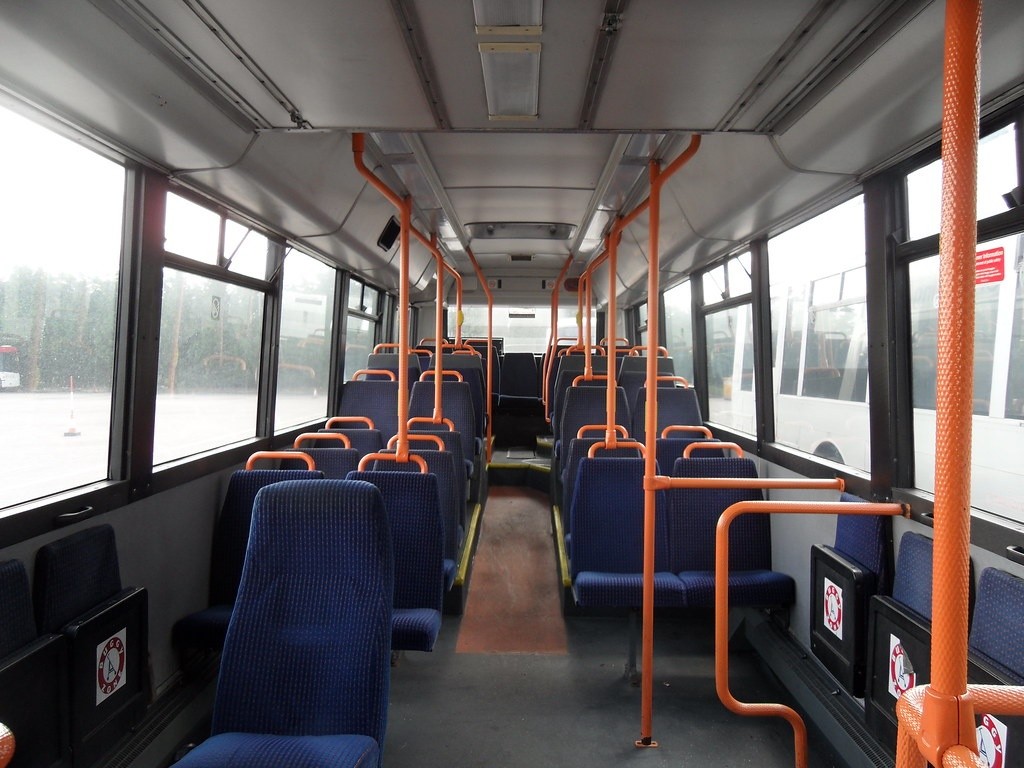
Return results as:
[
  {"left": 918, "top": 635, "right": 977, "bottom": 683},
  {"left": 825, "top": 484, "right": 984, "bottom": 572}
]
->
[
  {"left": 656, "top": 425, "right": 726, "bottom": 501},
  {"left": 338, "top": 369, "right": 398, "bottom": 448},
  {"left": 407, "top": 338, "right": 639, "bottom": 406},
  {"left": 372, "top": 433, "right": 458, "bottom": 591},
  {"left": 560, "top": 375, "right": 632, "bottom": 484},
  {"left": 428, "top": 344, "right": 485, "bottom": 455},
  {"left": 550, "top": 349, "right": 596, "bottom": 428},
  {"left": 366, "top": 343, "right": 420, "bottom": 401},
  {"left": 554, "top": 346, "right": 615, "bottom": 458},
  {"left": 632, "top": 376, "right": 704, "bottom": 446},
  {"left": 340, "top": 453, "right": 447, "bottom": 652},
  {"left": 313, "top": 416, "right": 381, "bottom": 470},
  {"left": 280, "top": 432, "right": 359, "bottom": 480},
  {"left": 617, "top": 345, "right": 675, "bottom": 421},
  {"left": 569, "top": 442, "right": 686, "bottom": 614},
  {"left": 565, "top": 424, "right": 641, "bottom": 558},
  {"left": 673, "top": 441, "right": 797, "bottom": 611},
  {"left": 174, "top": 482, "right": 395, "bottom": 768},
  {"left": 408, "top": 371, "right": 476, "bottom": 501},
  {"left": 389, "top": 417, "right": 467, "bottom": 546},
  {"left": 172, "top": 451, "right": 324, "bottom": 651}
]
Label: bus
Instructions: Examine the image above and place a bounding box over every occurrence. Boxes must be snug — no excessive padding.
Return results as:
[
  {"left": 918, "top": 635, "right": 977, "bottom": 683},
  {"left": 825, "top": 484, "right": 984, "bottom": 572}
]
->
[
  {"left": 727, "top": 186, "right": 1023, "bottom": 523},
  {"left": 0, "top": 344, "right": 21, "bottom": 388}
]
